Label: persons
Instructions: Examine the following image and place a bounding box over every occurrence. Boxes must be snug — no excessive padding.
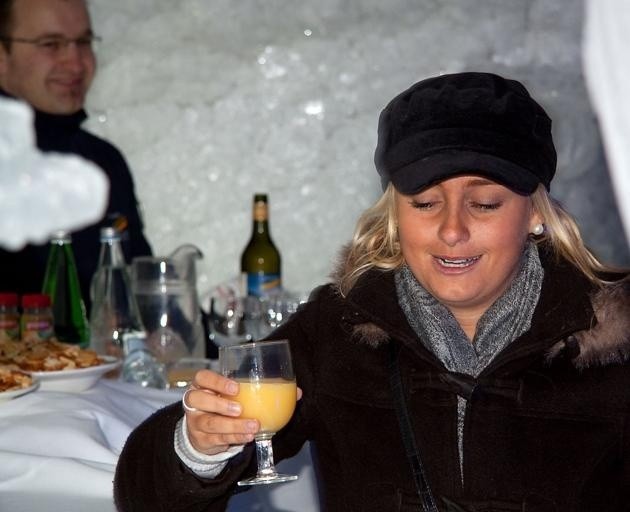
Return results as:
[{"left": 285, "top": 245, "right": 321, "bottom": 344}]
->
[
  {"left": 108, "top": 72, "right": 630, "bottom": 512},
  {"left": 1, "top": 0, "right": 239, "bottom": 366}
]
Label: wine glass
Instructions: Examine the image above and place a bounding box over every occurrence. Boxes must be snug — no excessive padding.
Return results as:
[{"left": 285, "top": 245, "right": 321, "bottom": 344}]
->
[{"left": 218, "top": 339, "right": 299, "bottom": 487}]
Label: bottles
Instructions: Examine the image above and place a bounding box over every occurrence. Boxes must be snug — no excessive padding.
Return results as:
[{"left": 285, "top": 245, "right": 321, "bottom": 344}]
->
[
  {"left": 120, "top": 244, "right": 207, "bottom": 393},
  {"left": 238, "top": 193, "right": 283, "bottom": 298},
  {"left": 39, "top": 229, "right": 90, "bottom": 349},
  {"left": 89, "top": 227, "right": 148, "bottom": 358}
]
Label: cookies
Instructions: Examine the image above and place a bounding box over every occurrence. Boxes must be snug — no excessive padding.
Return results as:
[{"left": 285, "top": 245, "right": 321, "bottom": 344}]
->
[{"left": 0, "top": 339, "right": 106, "bottom": 394}]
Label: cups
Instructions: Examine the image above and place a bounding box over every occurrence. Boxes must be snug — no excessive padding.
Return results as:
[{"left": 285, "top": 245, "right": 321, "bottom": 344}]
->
[{"left": 208, "top": 296, "right": 301, "bottom": 348}]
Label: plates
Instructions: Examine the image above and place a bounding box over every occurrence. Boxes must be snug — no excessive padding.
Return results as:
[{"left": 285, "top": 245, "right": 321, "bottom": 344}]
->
[
  {"left": 0, "top": 378, "right": 39, "bottom": 403},
  {"left": 24, "top": 354, "right": 122, "bottom": 393}
]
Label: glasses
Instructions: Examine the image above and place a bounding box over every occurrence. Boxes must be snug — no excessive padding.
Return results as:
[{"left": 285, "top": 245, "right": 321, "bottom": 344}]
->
[{"left": 9, "top": 34, "right": 102, "bottom": 54}]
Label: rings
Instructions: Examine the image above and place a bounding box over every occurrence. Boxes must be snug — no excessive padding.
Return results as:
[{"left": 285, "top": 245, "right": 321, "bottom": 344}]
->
[{"left": 183, "top": 388, "right": 196, "bottom": 412}]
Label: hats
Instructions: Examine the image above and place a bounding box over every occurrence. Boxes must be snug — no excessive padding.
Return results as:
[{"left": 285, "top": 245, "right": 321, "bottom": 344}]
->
[{"left": 374, "top": 72, "right": 558, "bottom": 198}]
